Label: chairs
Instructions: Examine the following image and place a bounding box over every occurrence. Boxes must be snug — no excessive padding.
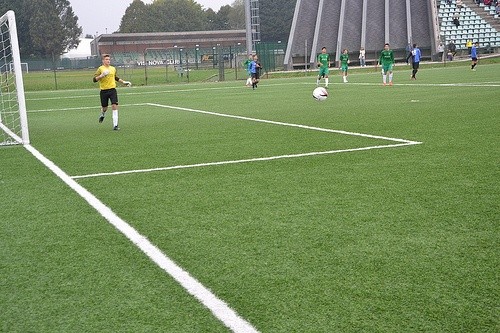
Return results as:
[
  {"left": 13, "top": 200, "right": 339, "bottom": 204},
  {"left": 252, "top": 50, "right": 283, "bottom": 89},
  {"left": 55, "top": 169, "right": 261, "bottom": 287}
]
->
[{"left": 438, "top": 0, "right": 500, "bottom": 50}]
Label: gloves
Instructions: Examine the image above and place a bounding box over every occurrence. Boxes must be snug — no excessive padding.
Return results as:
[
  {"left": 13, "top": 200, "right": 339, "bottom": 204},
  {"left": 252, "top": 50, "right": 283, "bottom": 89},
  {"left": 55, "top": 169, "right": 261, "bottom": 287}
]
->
[
  {"left": 100, "top": 71, "right": 109, "bottom": 78},
  {"left": 123, "top": 81, "right": 132, "bottom": 88}
]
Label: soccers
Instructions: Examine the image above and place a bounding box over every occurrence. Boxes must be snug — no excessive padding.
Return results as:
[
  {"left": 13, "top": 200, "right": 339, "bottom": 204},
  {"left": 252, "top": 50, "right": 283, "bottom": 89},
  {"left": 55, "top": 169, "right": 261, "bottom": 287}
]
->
[{"left": 311, "top": 86, "right": 328, "bottom": 102}]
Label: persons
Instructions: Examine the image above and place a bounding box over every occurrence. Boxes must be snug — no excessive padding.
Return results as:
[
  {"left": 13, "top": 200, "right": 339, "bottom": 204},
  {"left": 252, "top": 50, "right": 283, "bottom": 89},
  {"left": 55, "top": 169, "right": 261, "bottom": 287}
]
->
[
  {"left": 378, "top": 43, "right": 395, "bottom": 86},
  {"left": 448, "top": 40, "right": 457, "bottom": 56},
  {"left": 453, "top": 16, "right": 460, "bottom": 28},
  {"left": 467, "top": 39, "right": 472, "bottom": 55},
  {"left": 244, "top": 53, "right": 263, "bottom": 89},
  {"left": 471, "top": 42, "right": 478, "bottom": 71},
  {"left": 456, "top": 0, "right": 464, "bottom": 11},
  {"left": 317, "top": 47, "right": 330, "bottom": 89},
  {"left": 438, "top": 41, "right": 444, "bottom": 61},
  {"left": 358, "top": 47, "right": 366, "bottom": 67},
  {"left": 476, "top": 0, "right": 500, "bottom": 17},
  {"left": 406, "top": 44, "right": 421, "bottom": 80},
  {"left": 445, "top": 40, "right": 450, "bottom": 58},
  {"left": 93, "top": 54, "right": 132, "bottom": 130},
  {"left": 339, "top": 48, "right": 350, "bottom": 82}
]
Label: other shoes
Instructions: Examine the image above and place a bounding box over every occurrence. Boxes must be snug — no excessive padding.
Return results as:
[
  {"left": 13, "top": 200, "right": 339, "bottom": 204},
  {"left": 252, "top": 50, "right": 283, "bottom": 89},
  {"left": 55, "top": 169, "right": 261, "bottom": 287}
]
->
[
  {"left": 389, "top": 82, "right": 392, "bottom": 86},
  {"left": 344, "top": 80, "right": 348, "bottom": 83},
  {"left": 325, "top": 85, "right": 328, "bottom": 88},
  {"left": 255, "top": 84, "right": 257, "bottom": 88},
  {"left": 471, "top": 69, "right": 476, "bottom": 72},
  {"left": 411, "top": 77, "right": 416, "bottom": 80},
  {"left": 384, "top": 83, "right": 386, "bottom": 86}
]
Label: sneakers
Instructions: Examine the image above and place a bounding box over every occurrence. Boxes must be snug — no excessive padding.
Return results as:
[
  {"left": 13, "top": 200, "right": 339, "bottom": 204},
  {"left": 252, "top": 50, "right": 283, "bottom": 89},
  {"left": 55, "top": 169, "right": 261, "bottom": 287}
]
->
[
  {"left": 99, "top": 112, "right": 105, "bottom": 123},
  {"left": 114, "top": 125, "right": 120, "bottom": 130}
]
